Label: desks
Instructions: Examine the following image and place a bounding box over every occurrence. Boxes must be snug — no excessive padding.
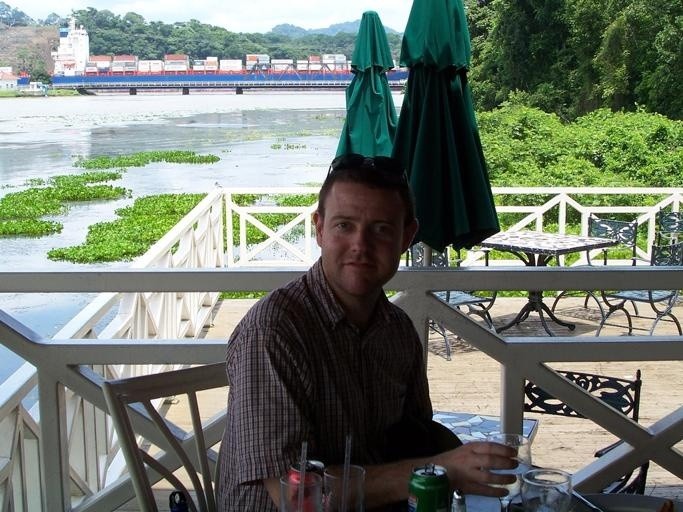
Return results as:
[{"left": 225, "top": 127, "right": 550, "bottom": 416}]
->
[
  {"left": 476, "top": 230, "right": 619, "bottom": 339},
  {"left": 433, "top": 407, "right": 539, "bottom": 450}
]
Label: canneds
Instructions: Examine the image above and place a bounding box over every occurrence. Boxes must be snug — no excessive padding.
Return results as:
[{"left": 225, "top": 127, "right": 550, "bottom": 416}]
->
[{"left": 407, "top": 463, "right": 450, "bottom": 512}]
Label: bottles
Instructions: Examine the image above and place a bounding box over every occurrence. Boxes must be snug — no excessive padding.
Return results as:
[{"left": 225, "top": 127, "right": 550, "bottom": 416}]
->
[{"left": 451, "top": 490, "right": 466, "bottom": 512}]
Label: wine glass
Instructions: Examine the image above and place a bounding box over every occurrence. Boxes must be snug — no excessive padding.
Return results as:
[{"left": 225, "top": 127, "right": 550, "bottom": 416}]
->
[{"left": 481, "top": 434, "right": 531, "bottom": 512}]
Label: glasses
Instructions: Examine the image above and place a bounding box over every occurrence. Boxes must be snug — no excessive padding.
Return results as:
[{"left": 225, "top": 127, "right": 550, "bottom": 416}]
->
[{"left": 328, "top": 154, "right": 406, "bottom": 179}]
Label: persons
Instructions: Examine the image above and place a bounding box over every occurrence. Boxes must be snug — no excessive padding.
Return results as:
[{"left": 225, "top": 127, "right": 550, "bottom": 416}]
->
[{"left": 214, "top": 155, "right": 519, "bottom": 512}]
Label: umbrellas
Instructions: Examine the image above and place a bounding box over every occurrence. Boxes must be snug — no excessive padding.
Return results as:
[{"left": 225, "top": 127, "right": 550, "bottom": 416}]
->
[
  {"left": 334, "top": 10, "right": 399, "bottom": 158},
  {"left": 389, "top": 0, "right": 503, "bottom": 265}
]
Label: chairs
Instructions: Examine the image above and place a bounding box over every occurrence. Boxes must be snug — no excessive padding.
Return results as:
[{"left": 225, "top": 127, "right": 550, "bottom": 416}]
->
[
  {"left": 98, "top": 362, "right": 229, "bottom": 512},
  {"left": 523, "top": 370, "right": 651, "bottom": 495},
  {"left": 407, "top": 243, "right": 499, "bottom": 364},
  {"left": 553, "top": 207, "right": 682, "bottom": 340}
]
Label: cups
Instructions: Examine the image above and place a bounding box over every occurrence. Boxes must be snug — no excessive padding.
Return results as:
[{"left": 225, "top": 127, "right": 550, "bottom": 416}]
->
[
  {"left": 325, "top": 464, "right": 365, "bottom": 511},
  {"left": 278, "top": 473, "right": 321, "bottom": 511},
  {"left": 521, "top": 469, "right": 572, "bottom": 511}
]
